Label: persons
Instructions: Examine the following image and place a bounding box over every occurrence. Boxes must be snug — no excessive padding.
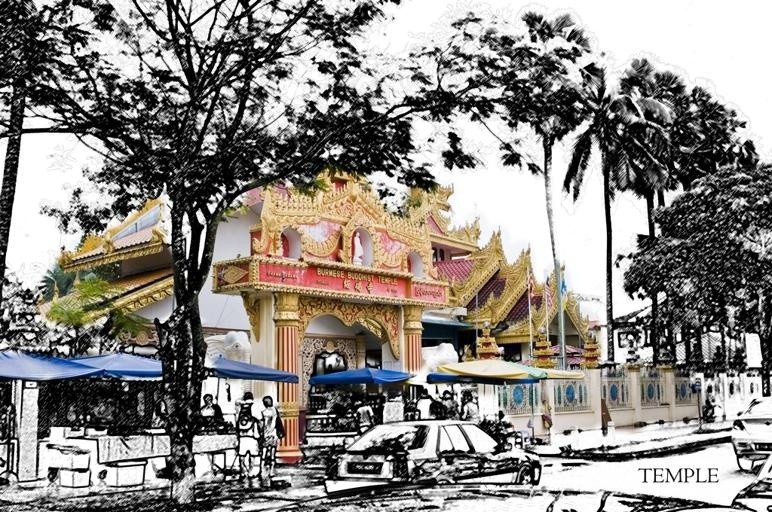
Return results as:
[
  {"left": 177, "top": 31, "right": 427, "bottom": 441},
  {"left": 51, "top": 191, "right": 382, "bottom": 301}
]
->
[
  {"left": 704, "top": 385, "right": 716, "bottom": 418},
  {"left": 66, "top": 390, "right": 278, "bottom": 486},
  {"left": 329, "top": 388, "right": 480, "bottom": 434},
  {"left": 353, "top": 232, "right": 364, "bottom": 262}
]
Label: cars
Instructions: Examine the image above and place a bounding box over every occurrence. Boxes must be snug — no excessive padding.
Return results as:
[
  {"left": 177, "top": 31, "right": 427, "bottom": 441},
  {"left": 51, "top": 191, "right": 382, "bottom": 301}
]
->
[
  {"left": 731, "top": 398, "right": 772, "bottom": 512},
  {"left": 338, "top": 420, "right": 541, "bottom": 493}
]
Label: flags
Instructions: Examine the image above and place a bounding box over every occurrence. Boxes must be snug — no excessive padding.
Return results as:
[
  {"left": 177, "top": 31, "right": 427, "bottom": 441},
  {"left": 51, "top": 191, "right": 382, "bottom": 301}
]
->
[
  {"left": 546, "top": 277, "right": 553, "bottom": 310},
  {"left": 529, "top": 271, "right": 535, "bottom": 298},
  {"left": 562, "top": 275, "right": 569, "bottom": 312}
]
